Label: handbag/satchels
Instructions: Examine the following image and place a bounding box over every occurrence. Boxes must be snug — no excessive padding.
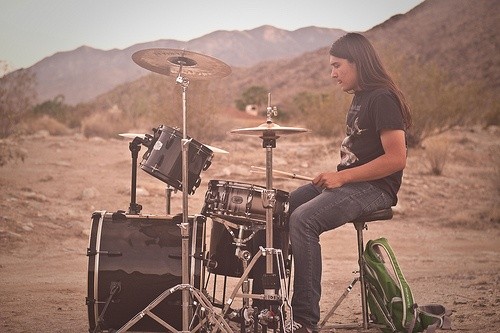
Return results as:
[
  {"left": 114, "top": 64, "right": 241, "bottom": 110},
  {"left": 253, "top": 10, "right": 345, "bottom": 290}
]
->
[{"left": 362, "top": 237, "right": 446, "bottom": 332}]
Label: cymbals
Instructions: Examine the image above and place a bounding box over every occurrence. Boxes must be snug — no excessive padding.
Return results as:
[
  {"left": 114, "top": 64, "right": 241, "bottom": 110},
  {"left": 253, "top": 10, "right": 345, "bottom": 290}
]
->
[
  {"left": 228, "top": 122, "right": 311, "bottom": 136},
  {"left": 118, "top": 131, "right": 228, "bottom": 155},
  {"left": 129, "top": 46, "right": 232, "bottom": 81}
]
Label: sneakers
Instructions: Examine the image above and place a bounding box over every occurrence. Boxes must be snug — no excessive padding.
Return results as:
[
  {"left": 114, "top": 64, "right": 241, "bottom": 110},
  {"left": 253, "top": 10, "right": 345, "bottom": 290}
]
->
[{"left": 275, "top": 320, "right": 316, "bottom": 332}]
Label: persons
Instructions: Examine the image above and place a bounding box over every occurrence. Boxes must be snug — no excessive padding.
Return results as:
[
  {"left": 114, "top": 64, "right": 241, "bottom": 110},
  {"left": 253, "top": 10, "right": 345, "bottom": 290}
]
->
[{"left": 227, "top": 33, "right": 412, "bottom": 333}]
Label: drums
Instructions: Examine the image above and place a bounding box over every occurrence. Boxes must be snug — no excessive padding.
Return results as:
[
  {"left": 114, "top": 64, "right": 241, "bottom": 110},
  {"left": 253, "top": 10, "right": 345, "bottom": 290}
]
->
[
  {"left": 85, "top": 210, "right": 206, "bottom": 333},
  {"left": 206, "top": 216, "right": 290, "bottom": 279},
  {"left": 200, "top": 179, "right": 290, "bottom": 227},
  {"left": 137, "top": 123, "right": 215, "bottom": 196}
]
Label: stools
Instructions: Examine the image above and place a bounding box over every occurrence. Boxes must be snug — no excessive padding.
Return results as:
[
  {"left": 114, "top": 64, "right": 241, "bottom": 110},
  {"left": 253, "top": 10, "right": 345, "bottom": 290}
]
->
[{"left": 319, "top": 208, "right": 394, "bottom": 333}]
